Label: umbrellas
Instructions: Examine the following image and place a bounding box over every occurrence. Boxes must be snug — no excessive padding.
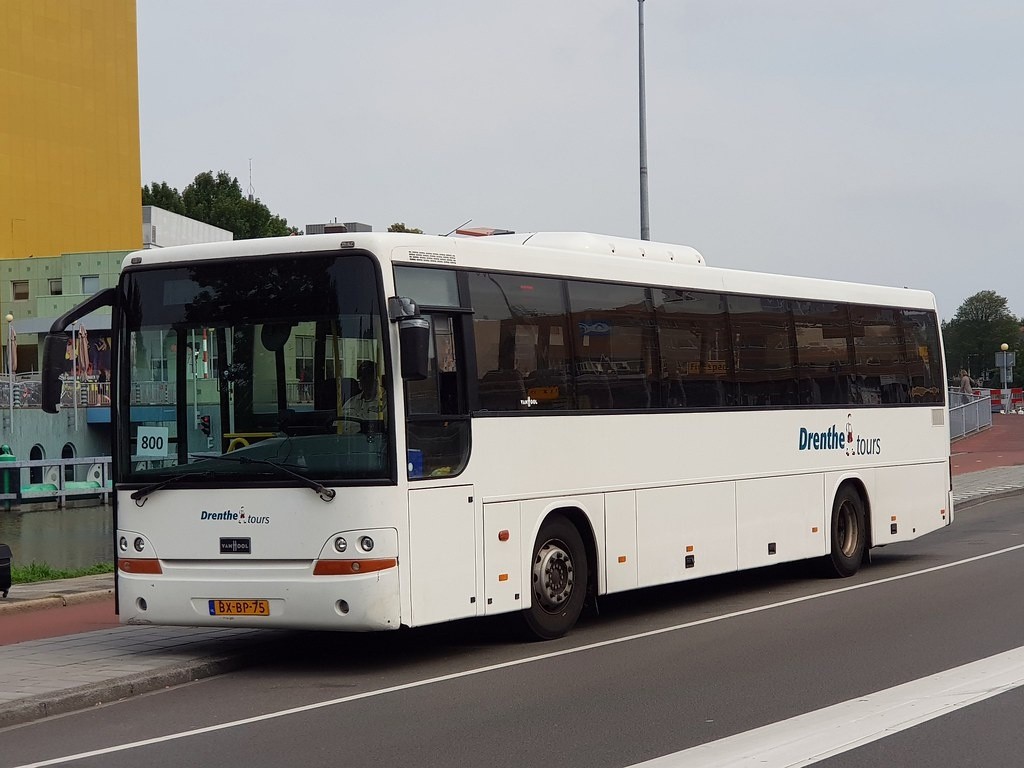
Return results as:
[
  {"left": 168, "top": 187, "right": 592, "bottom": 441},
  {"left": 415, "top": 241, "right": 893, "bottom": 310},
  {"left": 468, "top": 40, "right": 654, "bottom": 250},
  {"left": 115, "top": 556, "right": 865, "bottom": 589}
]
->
[
  {"left": 76, "top": 323, "right": 89, "bottom": 383},
  {"left": 7, "top": 325, "right": 17, "bottom": 375}
]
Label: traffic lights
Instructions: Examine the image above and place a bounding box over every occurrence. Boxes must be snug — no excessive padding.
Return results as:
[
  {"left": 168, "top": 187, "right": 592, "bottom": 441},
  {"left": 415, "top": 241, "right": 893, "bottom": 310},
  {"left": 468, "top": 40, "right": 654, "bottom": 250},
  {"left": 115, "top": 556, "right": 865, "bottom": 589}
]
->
[{"left": 200, "top": 416, "right": 210, "bottom": 435}]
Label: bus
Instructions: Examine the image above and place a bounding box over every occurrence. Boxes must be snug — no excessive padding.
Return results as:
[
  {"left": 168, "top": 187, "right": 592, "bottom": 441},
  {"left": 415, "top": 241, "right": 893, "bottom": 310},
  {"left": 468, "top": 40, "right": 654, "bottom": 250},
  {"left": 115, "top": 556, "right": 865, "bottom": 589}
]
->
[{"left": 39, "top": 231, "right": 956, "bottom": 642}]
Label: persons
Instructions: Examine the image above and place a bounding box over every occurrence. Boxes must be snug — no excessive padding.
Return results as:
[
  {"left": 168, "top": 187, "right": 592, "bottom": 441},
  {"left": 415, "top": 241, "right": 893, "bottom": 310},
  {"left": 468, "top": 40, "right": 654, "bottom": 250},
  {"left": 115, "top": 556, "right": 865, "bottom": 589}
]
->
[
  {"left": 341, "top": 361, "right": 388, "bottom": 419},
  {"left": 959, "top": 370, "right": 973, "bottom": 404},
  {"left": 94, "top": 337, "right": 107, "bottom": 374},
  {"left": 595, "top": 368, "right": 640, "bottom": 409},
  {"left": 296, "top": 367, "right": 311, "bottom": 403},
  {"left": 542, "top": 367, "right": 591, "bottom": 410},
  {"left": 94, "top": 369, "right": 110, "bottom": 406}
]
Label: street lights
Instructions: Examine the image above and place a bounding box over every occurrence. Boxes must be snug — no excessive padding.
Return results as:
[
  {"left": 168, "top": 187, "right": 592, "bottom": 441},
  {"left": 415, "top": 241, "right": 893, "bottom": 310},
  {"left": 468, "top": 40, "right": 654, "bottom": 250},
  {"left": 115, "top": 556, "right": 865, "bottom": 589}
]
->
[
  {"left": 1001, "top": 343, "right": 1008, "bottom": 412},
  {"left": 208, "top": 328, "right": 215, "bottom": 377},
  {"left": 71, "top": 320, "right": 79, "bottom": 431},
  {"left": 5, "top": 315, "right": 16, "bottom": 432}
]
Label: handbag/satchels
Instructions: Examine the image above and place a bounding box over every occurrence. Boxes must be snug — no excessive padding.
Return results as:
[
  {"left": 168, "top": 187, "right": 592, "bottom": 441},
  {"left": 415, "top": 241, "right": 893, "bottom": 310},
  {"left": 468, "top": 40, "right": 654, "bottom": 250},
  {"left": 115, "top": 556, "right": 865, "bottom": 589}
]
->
[{"left": 970, "top": 379, "right": 975, "bottom": 388}]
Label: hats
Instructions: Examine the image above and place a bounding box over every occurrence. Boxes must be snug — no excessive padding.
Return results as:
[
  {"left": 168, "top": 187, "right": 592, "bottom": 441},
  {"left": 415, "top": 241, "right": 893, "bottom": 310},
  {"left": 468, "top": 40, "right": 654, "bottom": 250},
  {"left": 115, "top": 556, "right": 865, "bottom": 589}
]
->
[{"left": 356, "top": 361, "right": 377, "bottom": 378}]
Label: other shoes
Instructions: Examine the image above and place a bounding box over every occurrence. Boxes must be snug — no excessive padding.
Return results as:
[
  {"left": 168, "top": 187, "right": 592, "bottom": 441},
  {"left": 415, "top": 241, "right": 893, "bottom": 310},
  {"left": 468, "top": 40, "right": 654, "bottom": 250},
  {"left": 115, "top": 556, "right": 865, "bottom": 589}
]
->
[{"left": 95, "top": 404, "right": 101, "bottom": 406}]
